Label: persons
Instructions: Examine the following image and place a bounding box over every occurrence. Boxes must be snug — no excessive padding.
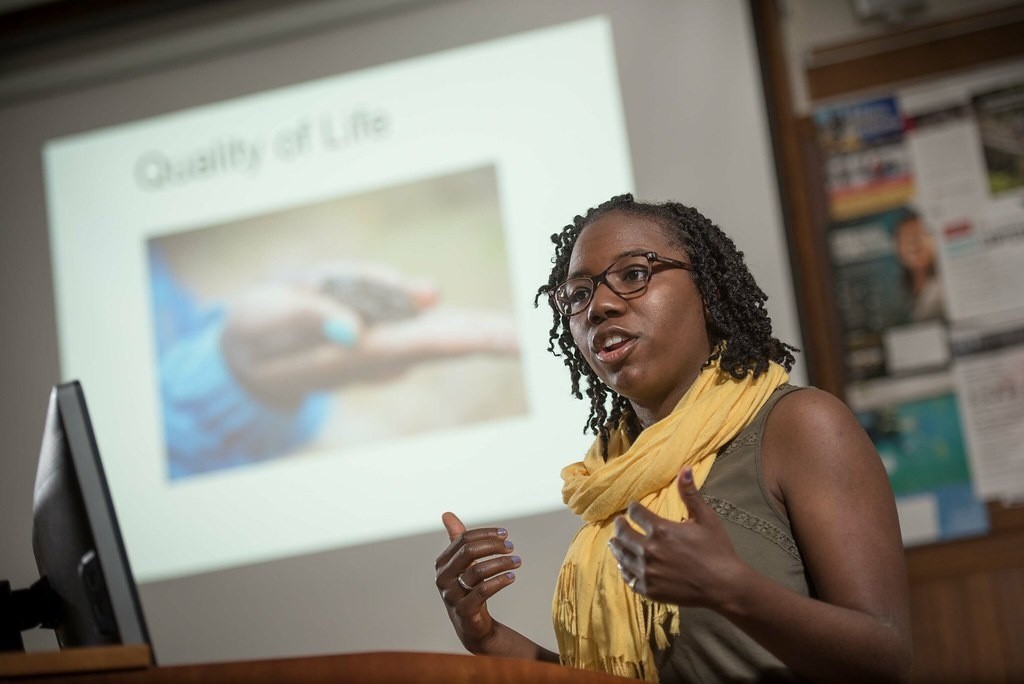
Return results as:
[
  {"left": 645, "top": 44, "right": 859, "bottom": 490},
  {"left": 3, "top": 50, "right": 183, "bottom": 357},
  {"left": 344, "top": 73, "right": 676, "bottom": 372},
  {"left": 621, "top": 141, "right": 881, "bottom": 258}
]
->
[
  {"left": 148, "top": 249, "right": 522, "bottom": 482},
  {"left": 434, "top": 195, "right": 922, "bottom": 684}
]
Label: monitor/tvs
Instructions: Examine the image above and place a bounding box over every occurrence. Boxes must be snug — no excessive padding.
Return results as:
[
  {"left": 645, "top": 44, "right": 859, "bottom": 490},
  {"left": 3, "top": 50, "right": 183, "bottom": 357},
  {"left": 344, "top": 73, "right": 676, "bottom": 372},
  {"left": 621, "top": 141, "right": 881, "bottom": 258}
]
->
[{"left": 0, "top": 380, "right": 158, "bottom": 663}]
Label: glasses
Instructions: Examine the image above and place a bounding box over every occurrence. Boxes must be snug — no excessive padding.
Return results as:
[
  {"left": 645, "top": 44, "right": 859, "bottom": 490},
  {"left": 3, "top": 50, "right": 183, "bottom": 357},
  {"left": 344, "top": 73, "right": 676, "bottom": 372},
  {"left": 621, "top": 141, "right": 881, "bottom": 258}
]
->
[{"left": 547, "top": 252, "right": 694, "bottom": 316}]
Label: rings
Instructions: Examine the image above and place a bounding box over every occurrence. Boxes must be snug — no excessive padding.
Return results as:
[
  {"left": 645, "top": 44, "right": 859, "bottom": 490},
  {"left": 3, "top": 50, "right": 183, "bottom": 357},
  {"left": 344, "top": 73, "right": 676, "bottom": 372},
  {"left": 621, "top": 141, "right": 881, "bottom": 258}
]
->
[
  {"left": 629, "top": 576, "right": 638, "bottom": 588},
  {"left": 458, "top": 574, "right": 474, "bottom": 590}
]
